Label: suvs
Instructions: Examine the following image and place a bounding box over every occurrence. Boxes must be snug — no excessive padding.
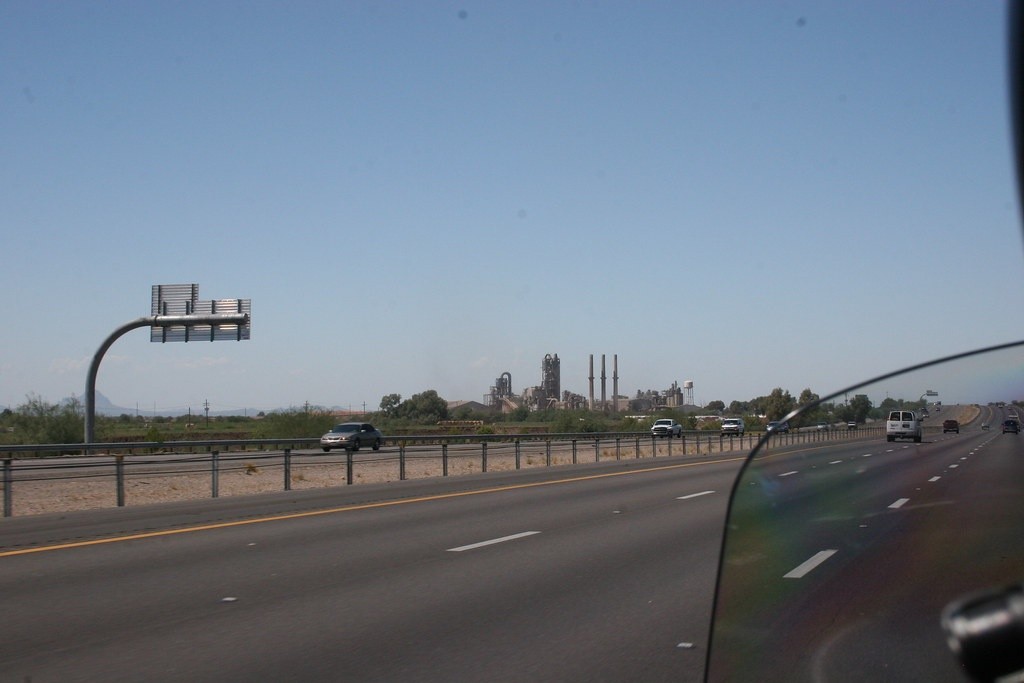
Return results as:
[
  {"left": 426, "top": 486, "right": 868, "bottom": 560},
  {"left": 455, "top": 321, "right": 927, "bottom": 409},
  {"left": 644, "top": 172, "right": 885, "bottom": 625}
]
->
[
  {"left": 720, "top": 417, "right": 744, "bottom": 436},
  {"left": 650, "top": 418, "right": 682, "bottom": 439}
]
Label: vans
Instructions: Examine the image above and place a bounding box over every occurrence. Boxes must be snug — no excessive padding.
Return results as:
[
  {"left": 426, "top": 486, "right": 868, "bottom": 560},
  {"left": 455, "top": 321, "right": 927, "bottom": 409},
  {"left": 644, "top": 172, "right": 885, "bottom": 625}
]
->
[{"left": 886, "top": 409, "right": 923, "bottom": 442}]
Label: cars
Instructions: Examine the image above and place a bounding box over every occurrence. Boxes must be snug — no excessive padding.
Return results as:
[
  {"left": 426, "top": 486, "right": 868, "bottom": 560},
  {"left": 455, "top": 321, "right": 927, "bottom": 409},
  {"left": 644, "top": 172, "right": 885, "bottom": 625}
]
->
[
  {"left": 848, "top": 421, "right": 857, "bottom": 429},
  {"left": 996, "top": 401, "right": 1021, "bottom": 435},
  {"left": 320, "top": 422, "right": 384, "bottom": 452},
  {"left": 981, "top": 422, "right": 989, "bottom": 430},
  {"left": 766, "top": 421, "right": 789, "bottom": 434},
  {"left": 935, "top": 407, "right": 940, "bottom": 411},
  {"left": 922, "top": 411, "right": 929, "bottom": 418},
  {"left": 816, "top": 421, "right": 828, "bottom": 432},
  {"left": 943, "top": 420, "right": 960, "bottom": 434}
]
